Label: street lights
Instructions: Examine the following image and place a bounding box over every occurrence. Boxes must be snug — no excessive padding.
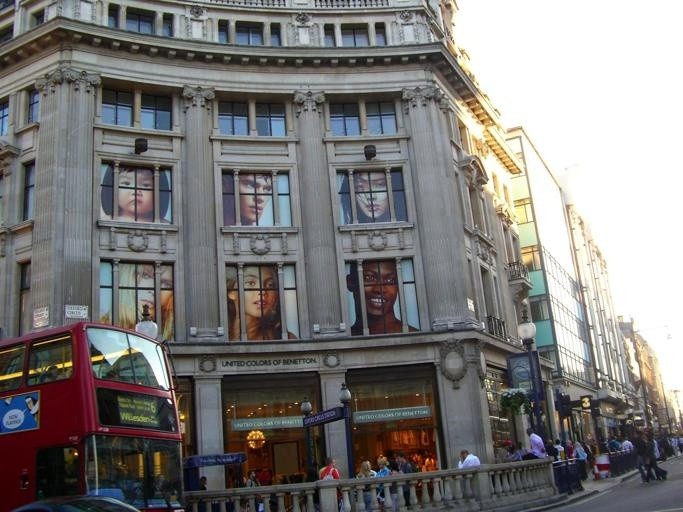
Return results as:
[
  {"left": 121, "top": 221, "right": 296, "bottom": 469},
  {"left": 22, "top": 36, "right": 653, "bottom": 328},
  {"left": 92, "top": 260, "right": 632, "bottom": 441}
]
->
[
  {"left": 298, "top": 398, "right": 316, "bottom": 481},
  {"left": 516, "top": 297, "right": 546, "bottom": 452},
  {"left": 337, "top": 382, "right": 354, "bottom": 479},
  {"left": 133, "top": 304, "right": 159, "bottom": 338}
]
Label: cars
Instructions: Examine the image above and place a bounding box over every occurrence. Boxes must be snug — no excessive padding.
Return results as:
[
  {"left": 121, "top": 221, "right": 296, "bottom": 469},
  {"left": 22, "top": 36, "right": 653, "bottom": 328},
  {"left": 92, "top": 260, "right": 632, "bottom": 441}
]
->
[{"left": 16, "top": 496, "right": 141, "bottom": 512}]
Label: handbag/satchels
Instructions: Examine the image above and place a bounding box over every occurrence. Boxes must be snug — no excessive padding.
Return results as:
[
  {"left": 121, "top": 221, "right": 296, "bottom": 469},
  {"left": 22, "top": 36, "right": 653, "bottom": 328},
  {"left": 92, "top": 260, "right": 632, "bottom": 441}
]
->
[{"left": 322, "top": 474, "right": 334, "bottom": 480}]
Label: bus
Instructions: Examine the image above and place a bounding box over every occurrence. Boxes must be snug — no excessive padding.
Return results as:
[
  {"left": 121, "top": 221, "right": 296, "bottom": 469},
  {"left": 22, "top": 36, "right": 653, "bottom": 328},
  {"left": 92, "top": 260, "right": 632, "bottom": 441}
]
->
[{"left": 0, "top": 322, "right": 184, "bottom": 511}]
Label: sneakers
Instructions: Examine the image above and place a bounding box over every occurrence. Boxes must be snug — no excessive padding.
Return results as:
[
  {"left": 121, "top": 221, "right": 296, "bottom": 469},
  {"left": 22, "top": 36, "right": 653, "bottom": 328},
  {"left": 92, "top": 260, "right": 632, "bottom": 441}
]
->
[{"left": 640, "top": 473, "right": 669, "bottom": 487}]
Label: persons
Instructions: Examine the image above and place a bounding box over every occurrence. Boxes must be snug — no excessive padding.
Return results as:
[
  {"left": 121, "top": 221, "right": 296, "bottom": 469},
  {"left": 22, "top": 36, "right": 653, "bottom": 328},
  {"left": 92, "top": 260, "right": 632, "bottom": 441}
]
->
[
  {"left": 357, "top": 458, "right": 392, "bottom": 511},
  {"left": 344, "top": 258, "right": 418, "bottom": 336},
  {"left": 225, "top": 262, "right": 297, "bottom": 341},
  {"left": 221, "top": 171, "right": 273, "bottom": 227},
  {"left": 98, "top": 262, "right": 173, "bottom": 341},
  {"left": 551, "top": 436, "right": 600, "bottom": 495},
  {"left": 457, "top": 449, "right": 480, "bottom": 469},
  {"left": 394, "top": 452, "right": 417, "bottom": 510},
  {"left": 98, "top": 162, "right": 170, "bottom": 224},
  {"left": 318, "top": 457, "right": 344, "bottom": 511},
  {"left": 200, "top": 476, "right": 208, "bottom": 491},
  {"left": 502, "top": 429, "right": 547, "bottom": 462},
  {"left": 600, "top": 426, "right": 683, "bottom": 484},
  {"left": 244, "top": 471, "right": 259, "bottom": 511},
  {"left": 338, "top": 169, "right": 409, "bottom": 224}
]
